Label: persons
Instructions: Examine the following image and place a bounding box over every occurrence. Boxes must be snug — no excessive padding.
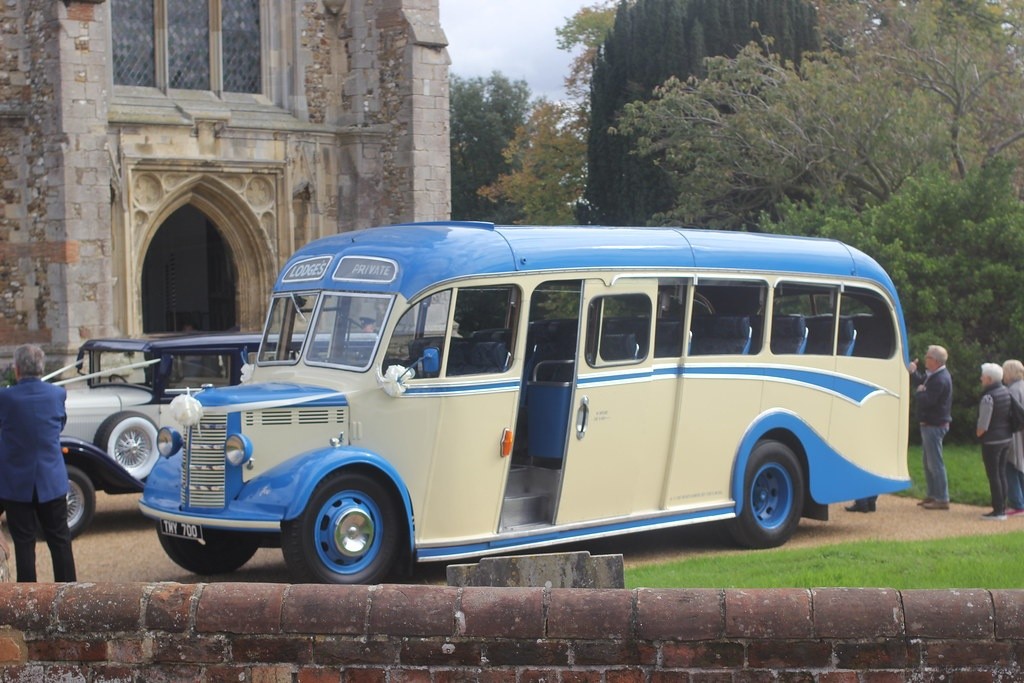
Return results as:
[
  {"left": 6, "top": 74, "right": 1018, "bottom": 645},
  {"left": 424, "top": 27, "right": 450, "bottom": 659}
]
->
[
  {"left": 0, "top": 343, "right": 77, "bottom": 582},
  {"left": 977, "top": 360, "right": 1024, "bottom": 520},
  {"left": 845, "top": 494, "right": 878, "bottom": 513},
  {"left": 359, "top": 317, "right": 380, "bottom": 335},
  {"left": 910, "top": 344, "right": 952, "bottom": 510}
]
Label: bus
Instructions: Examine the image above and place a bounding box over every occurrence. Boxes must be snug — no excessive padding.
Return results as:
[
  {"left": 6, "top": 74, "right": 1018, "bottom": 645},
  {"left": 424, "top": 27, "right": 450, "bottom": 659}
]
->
[{"left": 139, "top": 222, "right": 912, "bottom": 585}]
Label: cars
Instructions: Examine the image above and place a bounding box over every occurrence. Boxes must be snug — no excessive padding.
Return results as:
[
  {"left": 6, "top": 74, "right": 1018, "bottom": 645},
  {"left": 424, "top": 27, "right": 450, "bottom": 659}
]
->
[{"left": 37, "top": 333, "right": 379, "bottom": 540}]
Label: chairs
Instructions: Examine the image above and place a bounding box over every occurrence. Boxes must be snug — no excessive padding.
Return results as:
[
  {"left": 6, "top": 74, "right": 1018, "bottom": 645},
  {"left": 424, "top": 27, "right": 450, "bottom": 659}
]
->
[{"left": 409, "top": 315, "right": 857, "bottom": 369}]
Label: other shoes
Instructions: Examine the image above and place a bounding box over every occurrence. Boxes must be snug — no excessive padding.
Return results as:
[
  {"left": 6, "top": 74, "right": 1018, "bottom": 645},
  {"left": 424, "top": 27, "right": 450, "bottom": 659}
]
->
[
  {"left": 845, "top": 503, "right": 869, "bottom": 513},
  {"left": 981, "top": 509, "right": 1007, "bottom": 520},
  {"left": 917, "top": 497, "right": 935, "bottom": 506},
  {"left": 868, "top": 502, "right": 876, "bottom": 511},
  {"left": 1005, "top": 508, "right": 1024, "bottom": 516},
  {"left": 924, "top": 501, "right": 950, "bottom": 509}
]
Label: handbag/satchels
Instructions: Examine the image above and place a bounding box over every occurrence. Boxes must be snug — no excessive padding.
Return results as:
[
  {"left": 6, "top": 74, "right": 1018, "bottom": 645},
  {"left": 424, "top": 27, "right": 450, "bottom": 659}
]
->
[{"left": 1009, "top": 391, "right": 1024, "bottom": 433}]
